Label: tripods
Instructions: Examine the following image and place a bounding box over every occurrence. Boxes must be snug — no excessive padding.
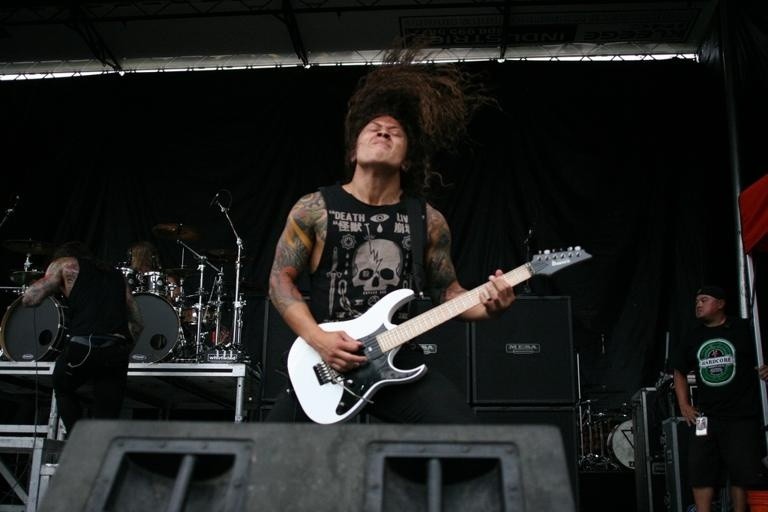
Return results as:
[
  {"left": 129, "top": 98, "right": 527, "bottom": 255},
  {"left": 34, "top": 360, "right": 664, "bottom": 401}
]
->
[{"left": 169, "top": 239, "right": 224, "bottom": 364}]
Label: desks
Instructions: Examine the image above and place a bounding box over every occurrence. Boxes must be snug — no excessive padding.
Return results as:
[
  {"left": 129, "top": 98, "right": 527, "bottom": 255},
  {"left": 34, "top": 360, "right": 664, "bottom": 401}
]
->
[{"left": 0, "top": 359, "right": 262, "bottom": 512}]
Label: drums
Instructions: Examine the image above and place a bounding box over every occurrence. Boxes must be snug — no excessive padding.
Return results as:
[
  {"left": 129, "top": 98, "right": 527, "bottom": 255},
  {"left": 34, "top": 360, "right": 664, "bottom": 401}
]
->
[
  {"left": 607, "top": 420, "right": 635, "bottom": 470},
  {"left": 181, "top": 309, "right": 216, "bottom": 333},
  {"left": 116, "top": 267, "right": 142, "bottom": 294},
  {"left": 1, "top": 293, "right": 70, "bottom": 363},
  {"left": 129, "top": 293, "right": 180, "bottom": 363},
  {"left": 142, "top": 271, "right": 166, "bottom": 296}
]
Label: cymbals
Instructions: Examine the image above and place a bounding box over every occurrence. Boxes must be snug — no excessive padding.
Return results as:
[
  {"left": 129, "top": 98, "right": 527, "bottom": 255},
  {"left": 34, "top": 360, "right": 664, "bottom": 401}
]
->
[{"left": 9, "top": 271, "right": 45, "bottom": 282}]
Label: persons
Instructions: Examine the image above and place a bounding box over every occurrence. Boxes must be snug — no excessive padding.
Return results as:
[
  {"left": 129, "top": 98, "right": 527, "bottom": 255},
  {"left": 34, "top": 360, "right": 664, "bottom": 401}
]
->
[
  {"left": 265, "top": 31, "right": 519, "bottom": 423},
  {"left": 21, "top": 239, "right": 145, "bottom": 440},
  {"left": 671, "top": 285, "right": 768, "bottom": 511}
]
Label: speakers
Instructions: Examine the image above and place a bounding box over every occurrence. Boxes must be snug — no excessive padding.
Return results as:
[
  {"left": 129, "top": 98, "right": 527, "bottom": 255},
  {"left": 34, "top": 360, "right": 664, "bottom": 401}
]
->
[
  {"left": 474, "top": 407, "right": 580, "bottom": 512},
  {"left": 471, "top": 295, "right": 575, "bottom": 404},
  {"left": 364, "top": 301, "right": 470, "bottom": 424},
  {"left": 34, "top": 419, "right": 576, "bottom": 512}
]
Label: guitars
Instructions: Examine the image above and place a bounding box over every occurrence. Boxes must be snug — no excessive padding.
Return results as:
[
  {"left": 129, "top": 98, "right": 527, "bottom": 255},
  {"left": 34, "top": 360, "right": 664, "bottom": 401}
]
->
[{"left": 288, "top": 246, "right": 594, "bottom": 426}]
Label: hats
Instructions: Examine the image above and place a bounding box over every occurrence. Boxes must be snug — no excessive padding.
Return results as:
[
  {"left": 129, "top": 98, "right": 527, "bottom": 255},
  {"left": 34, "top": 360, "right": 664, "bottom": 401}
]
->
[{"left": 692, "top": 285, "right": 725, "bottom": 301}]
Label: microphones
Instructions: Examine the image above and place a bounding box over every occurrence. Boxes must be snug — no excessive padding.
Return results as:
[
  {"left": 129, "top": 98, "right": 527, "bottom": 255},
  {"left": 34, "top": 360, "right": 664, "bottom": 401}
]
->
[
  {"left": 209, "top": 193, "right": 219, "bottom": 209},
  {"left": 176, "top": 224, "right": 183, "bottom": 245}
]
